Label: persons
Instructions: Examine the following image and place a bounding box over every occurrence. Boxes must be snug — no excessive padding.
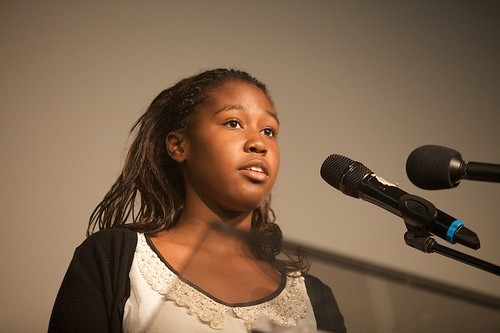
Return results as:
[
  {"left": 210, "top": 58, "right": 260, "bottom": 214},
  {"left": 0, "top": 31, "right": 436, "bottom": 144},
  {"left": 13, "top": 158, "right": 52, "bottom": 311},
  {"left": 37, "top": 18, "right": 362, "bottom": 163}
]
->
[{"left": 47, "top": 68, "right": 348, "bottom": 333}]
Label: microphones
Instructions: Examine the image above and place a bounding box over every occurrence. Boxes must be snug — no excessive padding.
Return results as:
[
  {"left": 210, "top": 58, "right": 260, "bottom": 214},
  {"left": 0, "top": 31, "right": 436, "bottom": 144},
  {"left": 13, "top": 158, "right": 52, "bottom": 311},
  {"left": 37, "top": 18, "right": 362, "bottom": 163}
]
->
[
  {"left": 405, "top": 144, "right": 500, "bottom": 190},
  {"left": 320, "top": 154, "right": 480, "bottom": 250}
]
